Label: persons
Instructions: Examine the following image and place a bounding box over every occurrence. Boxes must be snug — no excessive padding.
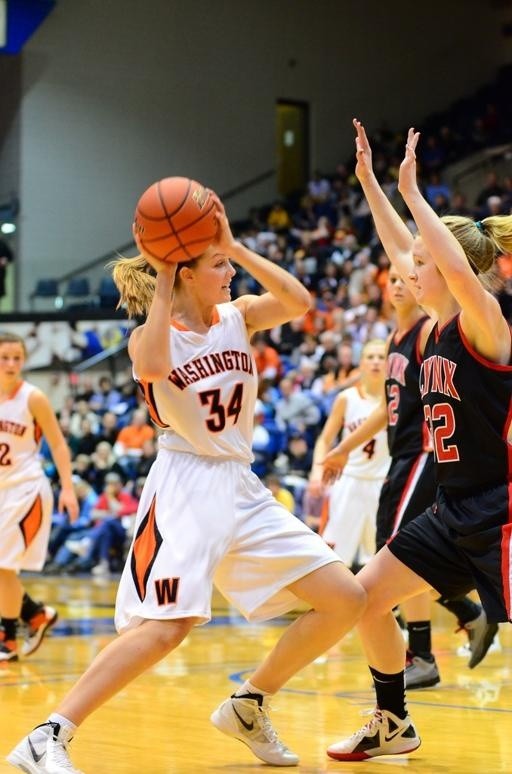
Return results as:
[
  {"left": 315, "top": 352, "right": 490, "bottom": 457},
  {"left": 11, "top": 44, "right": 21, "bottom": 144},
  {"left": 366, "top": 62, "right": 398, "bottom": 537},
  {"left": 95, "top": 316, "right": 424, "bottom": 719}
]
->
[
  {"left": 1, "top": 330, "right": 83, "bottom": 667},
  {"left": 2, "top": 132, "right": 512, "bottom": 580},
  {"left": 316, "top": 263, "right": 500, "bottom": 689},
  {"left": 1, "top": 185, "right": 372, "bottom": 774},
  {"left": 327, "top": 117, "right": 512, "bottom": 760}
]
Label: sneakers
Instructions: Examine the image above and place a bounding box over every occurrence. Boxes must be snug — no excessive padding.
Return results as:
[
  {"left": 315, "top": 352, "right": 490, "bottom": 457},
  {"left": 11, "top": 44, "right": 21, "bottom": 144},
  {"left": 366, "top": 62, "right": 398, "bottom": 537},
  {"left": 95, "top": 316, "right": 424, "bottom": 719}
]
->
[
  {"left": 326, "top": 708, "right": 421, "bottom": 761},
  {"left": 457, "top": 604, "right": 499, "bottom": 668},
  {"left": 210, "top": 693, "right": 298, "bottom": 766},
  {"left": 5, "top": 726, "right": 85, "bottom": 774},
  {"left": 0, "top": 605, "right": 58, "bottom": 661},
  {"left": 404, "top": 650, "right": 440, "bottom": 688}
]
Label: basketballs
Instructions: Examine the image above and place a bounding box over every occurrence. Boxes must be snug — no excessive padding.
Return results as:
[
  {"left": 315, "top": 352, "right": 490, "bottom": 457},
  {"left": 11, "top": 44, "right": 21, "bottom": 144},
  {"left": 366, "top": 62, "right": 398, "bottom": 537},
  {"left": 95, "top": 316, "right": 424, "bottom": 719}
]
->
[{"left": 133, "top": 175, "right": 219, "bottom": 263}]
